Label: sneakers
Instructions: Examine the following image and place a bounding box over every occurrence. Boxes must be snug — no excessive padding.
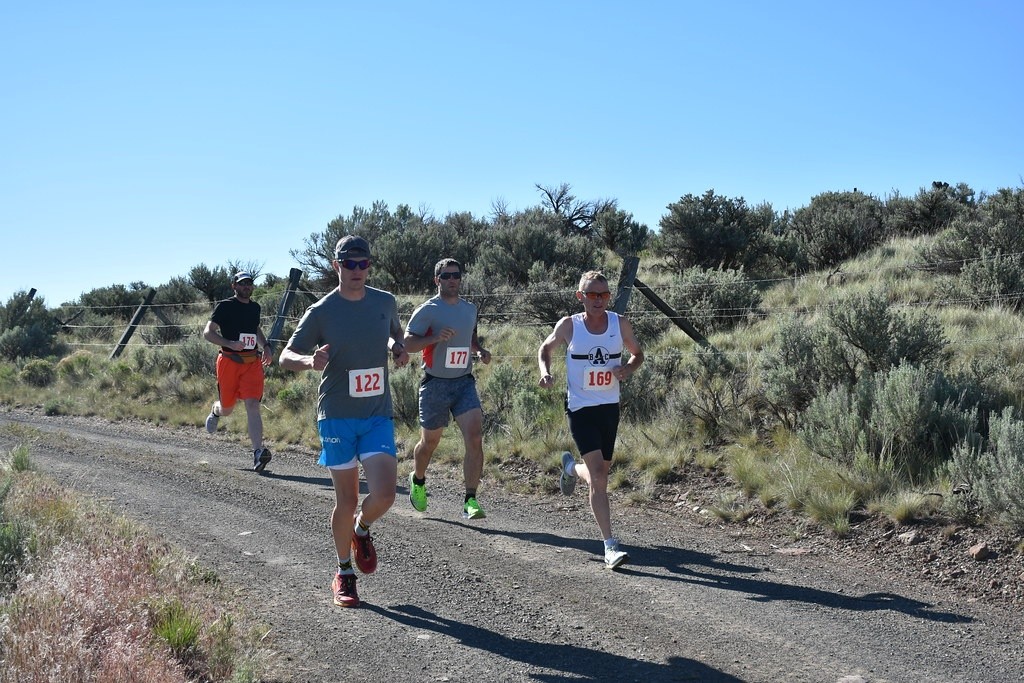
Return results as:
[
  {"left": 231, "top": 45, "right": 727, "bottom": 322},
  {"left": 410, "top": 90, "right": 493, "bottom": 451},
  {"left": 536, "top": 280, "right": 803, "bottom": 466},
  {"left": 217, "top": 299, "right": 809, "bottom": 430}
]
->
[
  {"left": 206, "top": 401, "right": 220, "bottom": 433},
  {"left": 560, "top": 451, "right": 576, "bottom": 497},
  {"left": 350, "top": 515, "right": 377, "bottom": 573},
  {"left": 604, "top": 544, "right": 630, "bottom": 569},
  {"left": 332, "top": 571, "right": 360, "bottom": 606},
  {"left": 464, "top": 497, "right": 486, "bottom": 520},
  {"left": 253, "top": 448, "right": 272, "bottom": 471},
  {"left": 409, "top": 471, "right": 427, "bottom": 512}
]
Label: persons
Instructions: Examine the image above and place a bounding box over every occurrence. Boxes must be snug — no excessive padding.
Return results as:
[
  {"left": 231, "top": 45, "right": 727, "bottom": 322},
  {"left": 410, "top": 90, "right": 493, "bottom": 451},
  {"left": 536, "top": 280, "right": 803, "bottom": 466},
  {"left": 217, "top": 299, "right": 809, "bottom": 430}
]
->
[
  {"left": 404, "top": 259, "right": 491, "bottom": 519},
  {"left": 537, "top": 271, "right": 644, "bottom": 570},
  {"left": 203, "top": 272, "right": 272, "bottom": 472},
  {"left": 279, "top": 235, "right": 409, "bottom": 607}
]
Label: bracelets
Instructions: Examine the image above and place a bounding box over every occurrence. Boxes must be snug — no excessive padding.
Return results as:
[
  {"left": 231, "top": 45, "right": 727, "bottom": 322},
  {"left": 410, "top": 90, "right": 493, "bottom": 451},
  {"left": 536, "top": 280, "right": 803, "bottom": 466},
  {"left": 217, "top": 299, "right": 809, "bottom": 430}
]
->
[
  {"left": 395, "top": 342, "right": 403, "bottom": 347},
  {"left": 264, "top": 342, "right": 273, "bottom": 349}
]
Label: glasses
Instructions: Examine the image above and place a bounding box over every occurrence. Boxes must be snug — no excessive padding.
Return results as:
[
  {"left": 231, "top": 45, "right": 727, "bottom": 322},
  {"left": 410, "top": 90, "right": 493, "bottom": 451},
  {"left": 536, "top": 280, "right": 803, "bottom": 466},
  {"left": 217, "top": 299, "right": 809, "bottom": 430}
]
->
[
  {"left": 337, "top": 259, "right": 370, "bottom": 270},
  {"left": 438, "top": 272, "right": 461, "bottom": 280},
  {"left": 581, "top": 290, "right": 610, "bottom": 300},
  {"left": 235, "top": 280, "right": 253, "bottom": 286}
]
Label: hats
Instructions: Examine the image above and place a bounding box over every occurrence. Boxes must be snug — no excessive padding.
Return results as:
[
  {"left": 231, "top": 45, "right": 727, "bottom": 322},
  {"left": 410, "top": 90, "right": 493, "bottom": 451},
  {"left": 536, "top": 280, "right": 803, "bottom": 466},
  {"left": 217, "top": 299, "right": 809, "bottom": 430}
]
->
[
  {"left": 234, "top": 272, "right": 253, "bottom": 284},
  {"left": 335, "top": 234, "right": 370, "bottom": 259}
]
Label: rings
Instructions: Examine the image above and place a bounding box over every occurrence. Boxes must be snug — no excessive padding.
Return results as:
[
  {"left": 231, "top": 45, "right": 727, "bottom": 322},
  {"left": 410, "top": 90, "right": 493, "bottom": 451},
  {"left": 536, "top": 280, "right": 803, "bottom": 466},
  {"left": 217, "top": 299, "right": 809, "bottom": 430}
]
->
[
  {"left": 620, "top": 375, "right": 623, "bottom": 377},
  {"left": 402, "top": 362, "right": 404, "bottom": 365}
]
